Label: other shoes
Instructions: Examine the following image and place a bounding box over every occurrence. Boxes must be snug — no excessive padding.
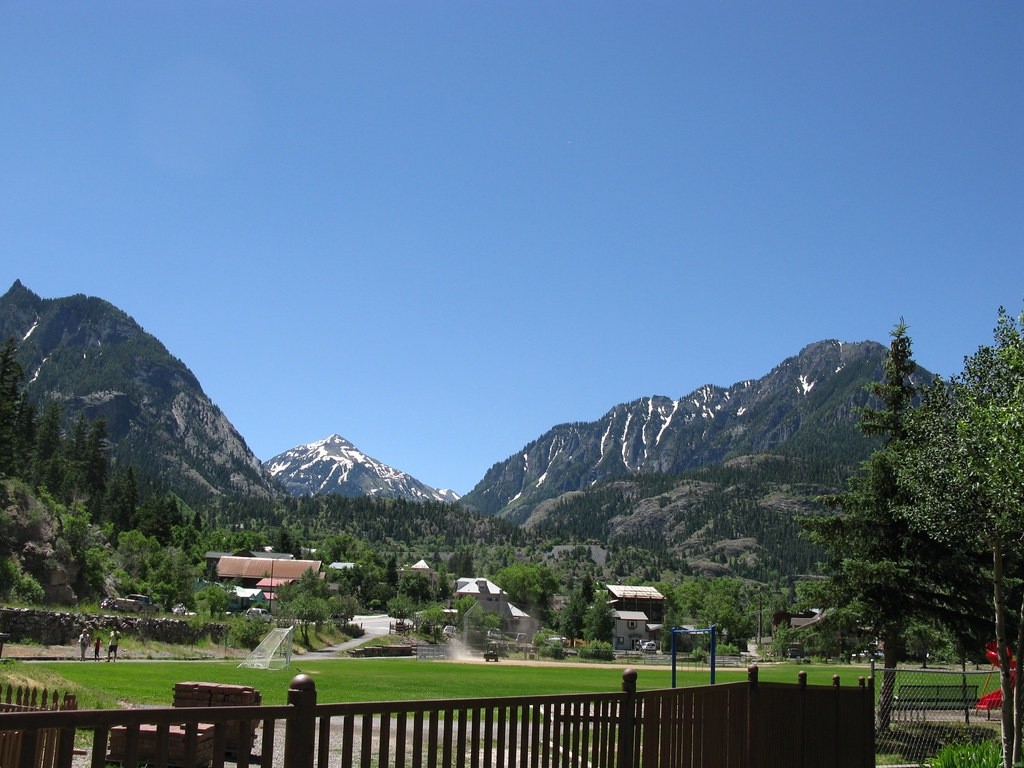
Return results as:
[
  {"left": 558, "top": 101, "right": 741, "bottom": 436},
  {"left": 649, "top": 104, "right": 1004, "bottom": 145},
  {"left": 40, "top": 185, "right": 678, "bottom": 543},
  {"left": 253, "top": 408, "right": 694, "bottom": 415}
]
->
[
  {"left": 83, "top": 659, "right": 85, "bottom": 661},
  {"left": 105, "top": 660, "right": 109, "bottom": 662},
  {"left": 80, "top": 658, "right": 82, "bottom": 661}
]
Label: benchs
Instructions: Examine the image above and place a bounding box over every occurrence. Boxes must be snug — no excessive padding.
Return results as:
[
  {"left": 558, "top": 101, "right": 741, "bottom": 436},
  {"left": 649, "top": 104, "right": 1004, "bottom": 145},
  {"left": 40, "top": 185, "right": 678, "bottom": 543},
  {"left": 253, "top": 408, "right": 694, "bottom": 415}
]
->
[{"left": 879, "top": 685, "right": 980, "bottom": 722}]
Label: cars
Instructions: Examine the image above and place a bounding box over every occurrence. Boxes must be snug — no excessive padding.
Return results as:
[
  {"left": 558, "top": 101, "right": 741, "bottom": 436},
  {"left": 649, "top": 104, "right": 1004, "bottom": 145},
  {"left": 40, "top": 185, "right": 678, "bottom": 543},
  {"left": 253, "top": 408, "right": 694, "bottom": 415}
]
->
[
  {"left": 246, "top": 608, "right": 271, "bottom": 619},
  {"left": 642, "top": 642, "right": 656, "bottom": 651},
  {"left": 443, "top": 626, "right": 456, "bottom": 633},
  {"left": 116, "top": 594, "right": 159, "bottom": 614}
]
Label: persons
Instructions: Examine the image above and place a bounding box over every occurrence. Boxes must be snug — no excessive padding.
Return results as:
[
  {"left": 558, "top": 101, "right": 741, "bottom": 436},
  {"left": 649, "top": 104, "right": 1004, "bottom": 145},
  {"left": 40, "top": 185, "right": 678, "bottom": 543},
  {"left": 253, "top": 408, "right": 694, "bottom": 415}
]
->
[
  {"left": 106, "top": 626, "right": 121, "bottom": 662},
  {"left": 78, "top": 629, "right": 92, "bottom": 661},
  {"left": 94, "top": 638, "right": 102, "bottom": 661},
  {"left": 172, "top": 602, "right": 185, "bottom": 615},
  {"left": 105, "top": 596, "right": 116, "bottom": 610}
]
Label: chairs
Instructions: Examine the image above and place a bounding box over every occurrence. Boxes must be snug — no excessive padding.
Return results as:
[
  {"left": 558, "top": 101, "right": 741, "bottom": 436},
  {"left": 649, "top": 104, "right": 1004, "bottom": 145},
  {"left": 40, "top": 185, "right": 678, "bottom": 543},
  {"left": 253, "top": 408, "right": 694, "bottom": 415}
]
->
[{"left": 975, "top": 689, "right": 1002, "bottom": 720}]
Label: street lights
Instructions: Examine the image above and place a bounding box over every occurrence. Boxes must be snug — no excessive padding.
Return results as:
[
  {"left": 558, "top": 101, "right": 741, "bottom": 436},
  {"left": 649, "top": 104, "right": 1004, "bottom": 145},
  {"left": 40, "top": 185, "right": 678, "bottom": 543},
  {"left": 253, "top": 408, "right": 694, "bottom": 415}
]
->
[{"left": 265, "top": 548, "right": 293, "bottom": 615}]
